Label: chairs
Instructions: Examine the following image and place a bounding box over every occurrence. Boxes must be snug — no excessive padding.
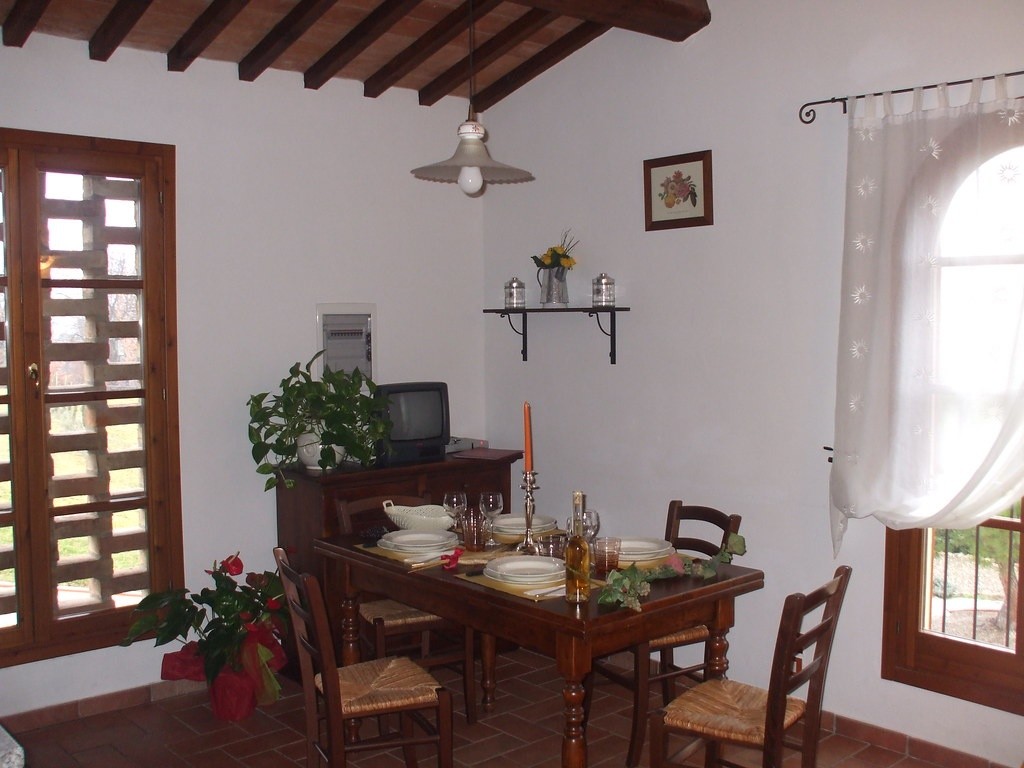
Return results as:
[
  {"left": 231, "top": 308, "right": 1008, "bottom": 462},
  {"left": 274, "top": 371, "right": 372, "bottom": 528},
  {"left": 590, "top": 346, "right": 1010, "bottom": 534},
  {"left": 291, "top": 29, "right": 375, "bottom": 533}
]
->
[
  {"left": 273, "top": 548, "right": 454, "bottom": 768},
  {"left": 583, "top": 499, "right": 741, "bottom": 768},
  {"left": 650, "top": 565, "right": 853, "bottom": 768},
  {"left": 332, "top": 495, "right": 477, "bottom": 723}
]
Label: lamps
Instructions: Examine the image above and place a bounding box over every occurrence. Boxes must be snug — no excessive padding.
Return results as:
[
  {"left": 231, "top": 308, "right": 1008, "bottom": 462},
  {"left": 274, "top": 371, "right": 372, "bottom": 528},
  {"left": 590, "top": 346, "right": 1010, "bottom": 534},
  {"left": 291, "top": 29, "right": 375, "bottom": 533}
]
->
[{"left": 409, "top": 18, "right": 532, "bottom": 191}]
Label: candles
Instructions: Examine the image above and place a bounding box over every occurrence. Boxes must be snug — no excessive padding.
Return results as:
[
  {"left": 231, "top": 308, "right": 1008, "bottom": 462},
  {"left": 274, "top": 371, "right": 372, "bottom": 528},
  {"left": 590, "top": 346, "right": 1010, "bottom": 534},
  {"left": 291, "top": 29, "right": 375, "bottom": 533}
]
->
[{"left": 523, "top": 402, "right": 533, "bottom": 471}]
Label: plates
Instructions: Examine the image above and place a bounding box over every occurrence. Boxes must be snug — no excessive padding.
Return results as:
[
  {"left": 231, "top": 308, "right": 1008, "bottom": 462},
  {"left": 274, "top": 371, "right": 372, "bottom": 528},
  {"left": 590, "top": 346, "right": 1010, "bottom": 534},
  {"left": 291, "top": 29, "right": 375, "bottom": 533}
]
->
[
  {"left": 487, "top": 526, "right": 557, "bottom": 534},
  {"left": 618, "top": 548, "right": 675, "bottom": 561},
  {"left": 486, "top": 555, "right": 566, "bottom": 581},
  {"left": 493, "top": 514, "right": 556, "bottom": 532},
  {"left": 382, "top": 528, "right": 457, "bottom": 550},
  {"left": 377, "top": 539, "right": 459, "bottom": 554},
  {"left": 616, "top": 538, "right": 673, "bottom": 558},
  {"left": 483, "top": 568, "right": 565, "bottom": 585}
]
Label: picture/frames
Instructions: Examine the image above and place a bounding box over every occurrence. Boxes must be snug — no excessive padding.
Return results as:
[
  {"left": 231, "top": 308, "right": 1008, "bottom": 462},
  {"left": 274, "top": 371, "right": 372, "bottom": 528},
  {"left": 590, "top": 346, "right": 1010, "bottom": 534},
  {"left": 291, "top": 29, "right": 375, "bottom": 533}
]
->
[{"left": 644, "top": 150, "right": 715, "bottom": 231}]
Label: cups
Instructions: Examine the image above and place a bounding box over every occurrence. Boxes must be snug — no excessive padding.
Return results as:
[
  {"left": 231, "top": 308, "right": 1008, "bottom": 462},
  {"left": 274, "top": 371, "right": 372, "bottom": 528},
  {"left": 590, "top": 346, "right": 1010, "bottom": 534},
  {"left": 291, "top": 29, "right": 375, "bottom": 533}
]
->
[
  {"left": 567, "top": 517, "right": 592, "bottom": 545},
  {"left": 583, "top": 509, "right": 600, "bottom": 541},
  {"left": 592, "top": 537, "right": 621, "bottom": 578},
  {"left": 537, "top": 535, "right": 564, "bottom": 559},
  {"left": 459, "top": 509, "right": 487, "bottom": 551}
]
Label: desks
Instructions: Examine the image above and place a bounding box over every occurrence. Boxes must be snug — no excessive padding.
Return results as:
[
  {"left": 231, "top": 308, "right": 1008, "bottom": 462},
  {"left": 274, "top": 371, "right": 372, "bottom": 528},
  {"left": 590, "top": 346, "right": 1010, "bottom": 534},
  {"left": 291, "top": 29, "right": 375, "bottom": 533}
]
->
[{"left": 313, "top": 531, "right": 765, "bottom": 768}]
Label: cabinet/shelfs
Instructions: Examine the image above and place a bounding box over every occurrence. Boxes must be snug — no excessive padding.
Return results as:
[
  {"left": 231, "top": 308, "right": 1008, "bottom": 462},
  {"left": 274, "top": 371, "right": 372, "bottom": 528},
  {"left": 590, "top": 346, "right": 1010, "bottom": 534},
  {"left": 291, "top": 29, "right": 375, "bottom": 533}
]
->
[{"left": 277, "top": 448, "right": 522, "bottom": 577}]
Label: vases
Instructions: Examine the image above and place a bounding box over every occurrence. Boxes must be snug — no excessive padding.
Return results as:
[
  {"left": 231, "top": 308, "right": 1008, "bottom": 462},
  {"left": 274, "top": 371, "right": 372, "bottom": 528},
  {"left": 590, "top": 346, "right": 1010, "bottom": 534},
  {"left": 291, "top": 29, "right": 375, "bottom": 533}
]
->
[
  {"left": 205, "top": 665, "right": 258, "bottom": 722},
  {"left": 537, "top": 268, "right": 571, "bottom": 307}
]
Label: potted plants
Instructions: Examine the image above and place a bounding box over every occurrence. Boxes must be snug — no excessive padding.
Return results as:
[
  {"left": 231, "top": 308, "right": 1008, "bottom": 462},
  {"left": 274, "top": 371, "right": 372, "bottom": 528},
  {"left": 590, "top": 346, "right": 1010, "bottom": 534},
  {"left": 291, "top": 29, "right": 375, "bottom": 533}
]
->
[{"left": 245, "top": 348, "right": 393, "bottom": 493}]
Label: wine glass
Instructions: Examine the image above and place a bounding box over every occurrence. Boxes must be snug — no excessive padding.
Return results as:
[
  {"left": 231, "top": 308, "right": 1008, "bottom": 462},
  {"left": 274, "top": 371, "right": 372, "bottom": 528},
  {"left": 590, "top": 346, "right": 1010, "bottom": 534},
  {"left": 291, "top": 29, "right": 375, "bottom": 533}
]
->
[
  {"left": 480, "top": 492, "right": 503, "bottom": 546},
  {"left": 443, "top": 492, "right": 467, "bottom": 533}
]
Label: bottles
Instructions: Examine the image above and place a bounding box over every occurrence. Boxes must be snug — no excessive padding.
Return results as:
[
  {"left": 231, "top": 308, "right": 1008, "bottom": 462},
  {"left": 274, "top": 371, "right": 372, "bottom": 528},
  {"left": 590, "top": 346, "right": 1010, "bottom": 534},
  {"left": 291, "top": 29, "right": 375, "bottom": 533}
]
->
[{"left": 566, "top": 491, "right": 591, "bottom": 603}]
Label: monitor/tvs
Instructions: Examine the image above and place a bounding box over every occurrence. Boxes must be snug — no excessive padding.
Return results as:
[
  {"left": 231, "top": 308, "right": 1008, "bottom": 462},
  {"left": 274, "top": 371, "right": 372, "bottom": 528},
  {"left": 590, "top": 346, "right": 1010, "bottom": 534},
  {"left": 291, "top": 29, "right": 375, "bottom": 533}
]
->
[{"left": 373, "top": 382, "right": 450, "bottom": 467}]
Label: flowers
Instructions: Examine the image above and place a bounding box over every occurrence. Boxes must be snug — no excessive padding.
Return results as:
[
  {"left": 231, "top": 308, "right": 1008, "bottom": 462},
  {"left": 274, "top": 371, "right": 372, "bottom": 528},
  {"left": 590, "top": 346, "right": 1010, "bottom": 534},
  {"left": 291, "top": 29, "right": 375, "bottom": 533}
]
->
[
  {"left": 122, "top": 554, "right": 287, "bottom": 696},
  {"left": 532, "top": 227, "right": 581, "bottom": 273}
]
